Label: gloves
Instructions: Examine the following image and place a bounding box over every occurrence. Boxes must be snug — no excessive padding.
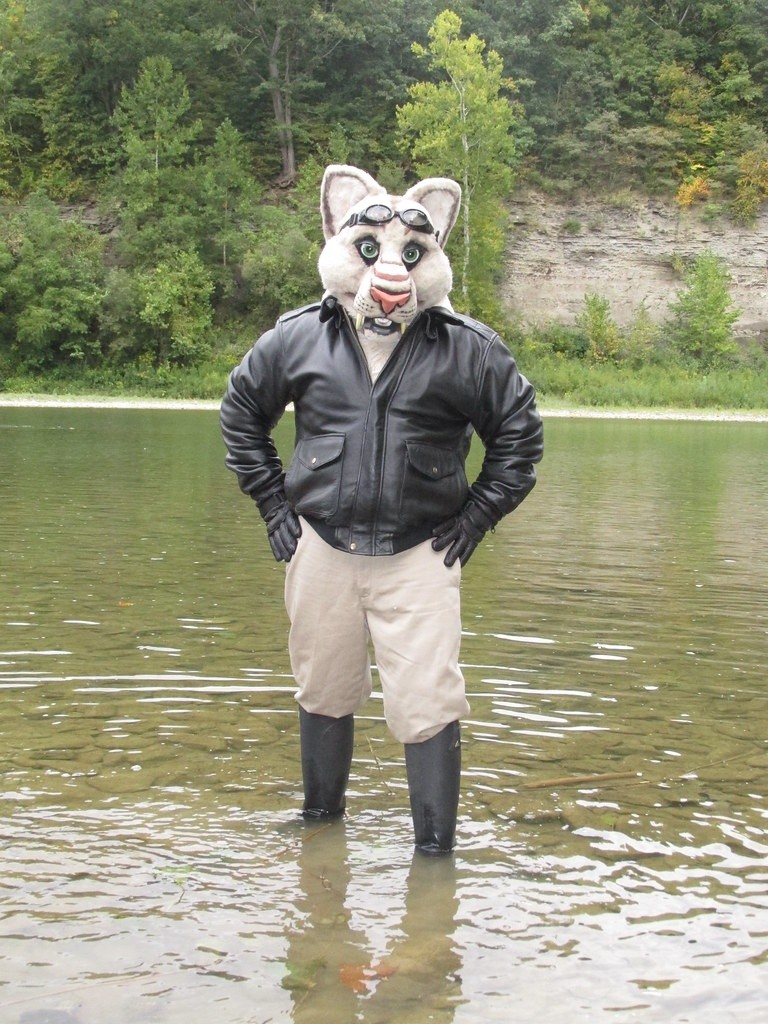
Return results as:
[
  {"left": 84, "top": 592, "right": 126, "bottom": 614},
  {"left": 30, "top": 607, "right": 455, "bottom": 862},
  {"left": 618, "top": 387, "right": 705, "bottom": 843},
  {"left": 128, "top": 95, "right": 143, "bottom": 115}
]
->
[
  {"left": 431, "top": 501, "right": 496, "bottom": 568},
  {"left": 263, "top": 501, "right": 302, "bottom": 562}
]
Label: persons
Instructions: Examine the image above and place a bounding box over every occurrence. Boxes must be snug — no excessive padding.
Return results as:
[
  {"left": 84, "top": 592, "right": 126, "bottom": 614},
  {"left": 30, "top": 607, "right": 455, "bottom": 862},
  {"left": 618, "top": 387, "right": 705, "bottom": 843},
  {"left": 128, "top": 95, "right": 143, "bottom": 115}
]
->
[{"left": 221, "top": 162, "right": 544, "bottom": 853}]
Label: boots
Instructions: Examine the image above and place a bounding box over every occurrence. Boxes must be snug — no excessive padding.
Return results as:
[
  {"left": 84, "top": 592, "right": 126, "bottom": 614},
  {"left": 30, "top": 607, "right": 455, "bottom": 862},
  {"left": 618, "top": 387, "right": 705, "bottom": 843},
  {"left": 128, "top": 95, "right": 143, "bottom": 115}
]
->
[
  {"left": 404, "top": 720, "right": 461, "bottom": 856},
  {"left": 298, "top": 704, "right": 354, "bottom": 823}
]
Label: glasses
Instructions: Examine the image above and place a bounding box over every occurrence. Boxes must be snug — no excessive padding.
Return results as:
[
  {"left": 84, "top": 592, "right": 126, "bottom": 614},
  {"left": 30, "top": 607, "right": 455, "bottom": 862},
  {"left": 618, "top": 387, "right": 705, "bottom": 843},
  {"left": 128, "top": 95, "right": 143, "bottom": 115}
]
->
[{"left": 336, "top": 204, "right": 437, "bottom": 234}]
